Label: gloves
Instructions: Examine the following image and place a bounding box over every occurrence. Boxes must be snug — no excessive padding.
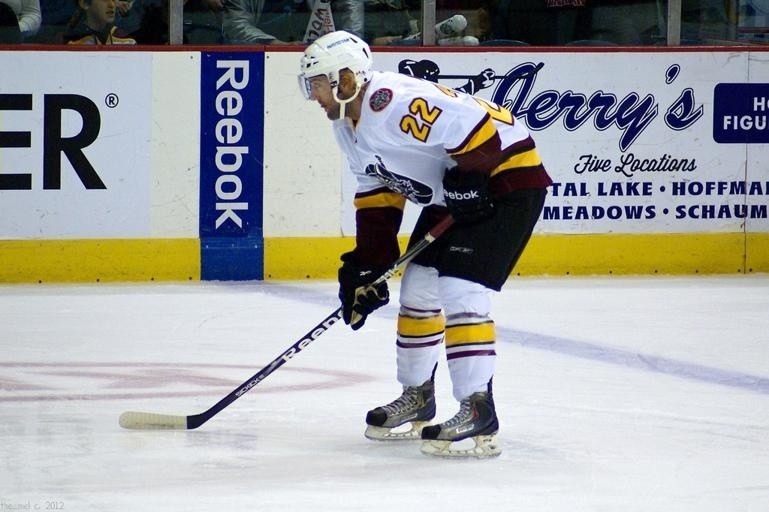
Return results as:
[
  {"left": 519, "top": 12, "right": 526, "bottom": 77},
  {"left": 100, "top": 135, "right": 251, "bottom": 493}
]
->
[
  {"left": 444, "top": 169, "right": 491, "bottom": 226},
  {"left": 338, "top": 260, "right": 391, "bottom": 331}
]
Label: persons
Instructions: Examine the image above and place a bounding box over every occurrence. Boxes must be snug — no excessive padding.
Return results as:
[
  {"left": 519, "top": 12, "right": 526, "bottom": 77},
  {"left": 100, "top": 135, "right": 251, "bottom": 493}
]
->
[
  {"left": 301, "top": 31, "right": 553, "bottom": 458},
  {"left": 0, "top": 0, "right": 769, "bottom": 46}
]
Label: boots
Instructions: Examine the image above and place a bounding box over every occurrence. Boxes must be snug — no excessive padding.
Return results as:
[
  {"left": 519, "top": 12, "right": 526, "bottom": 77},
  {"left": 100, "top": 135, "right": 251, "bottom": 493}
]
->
[
  {"left": 421, "top": 374, "right": 500, "bottom": 441},
  {"left": 366, "top": 359, "right": 438, "bottom": 427}
]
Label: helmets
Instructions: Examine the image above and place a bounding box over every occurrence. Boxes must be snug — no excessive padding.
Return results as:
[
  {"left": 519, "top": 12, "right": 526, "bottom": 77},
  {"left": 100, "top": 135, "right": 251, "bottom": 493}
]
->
[{"left": 298, "top": 31, "right": 373, "bottom": 100}]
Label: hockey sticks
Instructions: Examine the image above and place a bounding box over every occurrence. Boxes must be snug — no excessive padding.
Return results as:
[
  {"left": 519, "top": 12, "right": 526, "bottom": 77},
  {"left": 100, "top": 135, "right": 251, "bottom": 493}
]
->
[{"left": 118, "top": 215, "right": 456, "bottom": 429}]
